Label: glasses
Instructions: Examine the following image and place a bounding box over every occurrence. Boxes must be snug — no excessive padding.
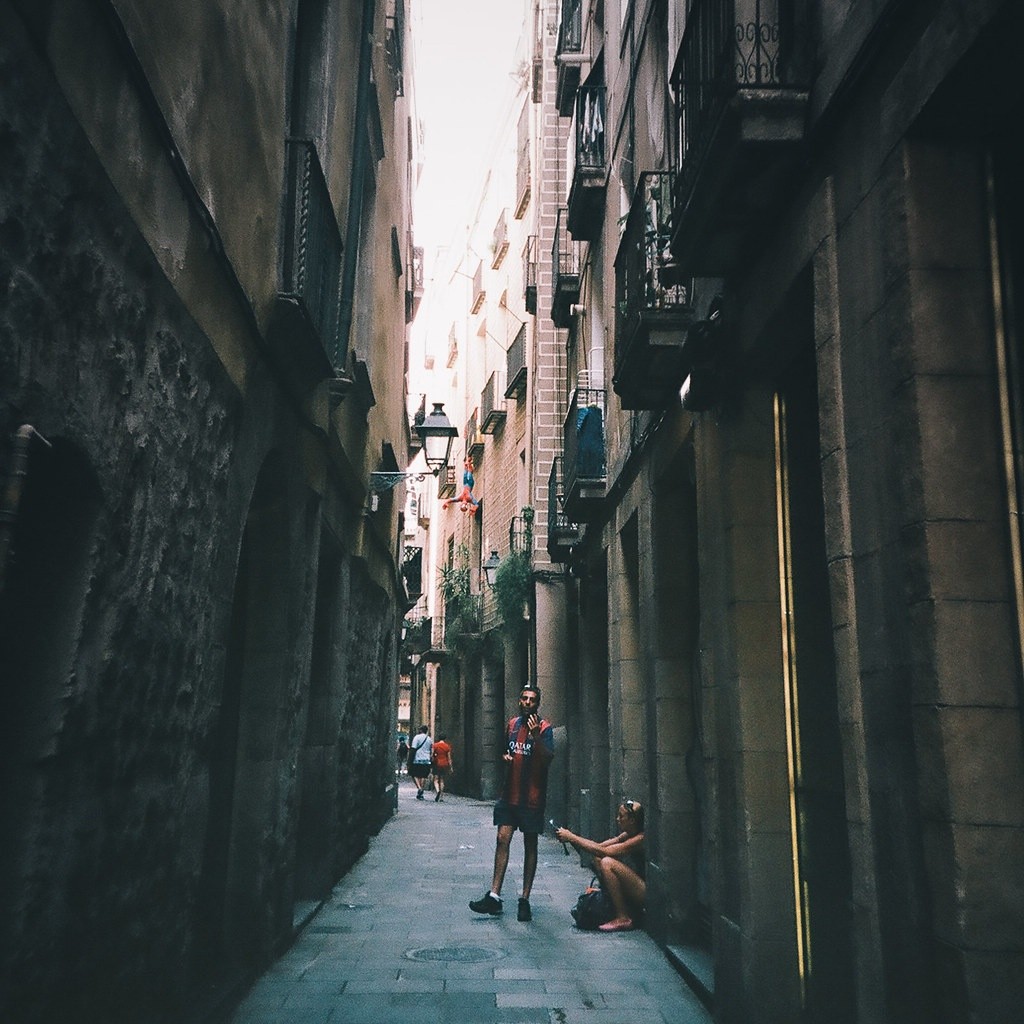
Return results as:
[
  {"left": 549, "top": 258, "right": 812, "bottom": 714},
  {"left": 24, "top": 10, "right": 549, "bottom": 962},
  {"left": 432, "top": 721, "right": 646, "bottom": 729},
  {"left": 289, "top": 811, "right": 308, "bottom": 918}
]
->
[
  {"left": 523, "top": 684, "right": 540, "bottom": 694},
  {"left": 623, "top": 801, "right": 637, "bottom": 822}
]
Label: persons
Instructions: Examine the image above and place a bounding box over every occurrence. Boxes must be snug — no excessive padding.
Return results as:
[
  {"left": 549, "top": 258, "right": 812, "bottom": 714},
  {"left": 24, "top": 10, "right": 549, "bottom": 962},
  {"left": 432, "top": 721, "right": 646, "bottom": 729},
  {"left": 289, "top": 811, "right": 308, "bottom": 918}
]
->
[
  {"left": 442, "top": 457, "right": 479, "bottom": 515},
  {"left": 409, "top": 725, "right": 434, "bottom": 800},
  {"left": 431, "top": 731, "right": 454, "bottom": 802},
  {"left": 556, "top": 800, "right": 648, "bottom": 933},
  {"left": 396, "top": 737, "right": 410, "bottom": 774},
  {"left": 468, "top": 685, "right": 553, "bottom": 921}
]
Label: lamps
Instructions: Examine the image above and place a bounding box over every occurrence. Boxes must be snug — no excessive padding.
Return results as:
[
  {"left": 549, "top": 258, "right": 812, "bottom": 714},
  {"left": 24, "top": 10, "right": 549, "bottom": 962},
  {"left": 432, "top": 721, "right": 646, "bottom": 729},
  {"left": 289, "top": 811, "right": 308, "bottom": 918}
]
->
[
  {"left": 482, "top": 549, "right": 501, "bottom": 590},
  {"left": 370, "top": 402, "right": 460, "bottom": 494}
]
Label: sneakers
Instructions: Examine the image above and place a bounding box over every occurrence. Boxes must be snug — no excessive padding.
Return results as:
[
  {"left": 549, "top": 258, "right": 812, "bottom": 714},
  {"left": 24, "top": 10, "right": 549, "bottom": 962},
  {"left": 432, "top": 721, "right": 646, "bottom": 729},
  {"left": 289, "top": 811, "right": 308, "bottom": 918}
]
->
[
  {"left": 516, "top": 897, "right": 532, "bottom": 921},
  {"left": 468, "top": 890, "right": 504, "bottom": 915}
]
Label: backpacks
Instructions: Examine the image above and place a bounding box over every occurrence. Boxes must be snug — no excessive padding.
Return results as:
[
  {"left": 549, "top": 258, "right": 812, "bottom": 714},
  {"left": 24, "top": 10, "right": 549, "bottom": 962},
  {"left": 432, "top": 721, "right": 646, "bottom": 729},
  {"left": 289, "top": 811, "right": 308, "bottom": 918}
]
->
[
  {"left": 398, "top": 743, "right": 408, "bottom": 756},
  {"left": 570, "top": 876, "right": 616, "bottom": 929}
]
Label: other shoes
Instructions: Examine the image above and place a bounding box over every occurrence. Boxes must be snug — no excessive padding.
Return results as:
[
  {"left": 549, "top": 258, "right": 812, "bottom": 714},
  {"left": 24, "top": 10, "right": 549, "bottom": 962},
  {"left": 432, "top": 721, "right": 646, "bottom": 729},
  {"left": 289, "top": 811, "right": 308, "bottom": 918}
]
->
[
  {"left": 599, "top": 919, "right": 632, "bottom": 932},
  {"left": 416, "top": 789, "right": 423, "bottom": 798},
  {"left": 420, "top": 796, "right": 424, "bottom": 800},
  {"left": 435, "top": 790, "right": 441, "bottom": 802},
  {"left": 439, "top": 799, "right": 443, "bottom": 803}
]
